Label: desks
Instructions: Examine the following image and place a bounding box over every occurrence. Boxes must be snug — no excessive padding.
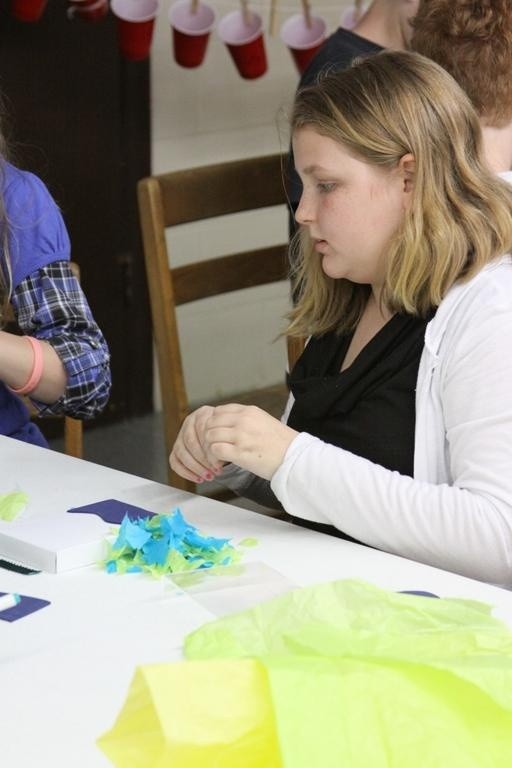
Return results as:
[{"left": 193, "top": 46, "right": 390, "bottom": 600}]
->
[{"left": 1, "top": 436, "right": 511, "bottom": 768}]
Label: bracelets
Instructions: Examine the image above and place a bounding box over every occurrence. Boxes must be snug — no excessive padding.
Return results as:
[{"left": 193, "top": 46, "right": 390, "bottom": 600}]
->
[{"left": 5, "top": 335, "right": 46, "bottom": 396}]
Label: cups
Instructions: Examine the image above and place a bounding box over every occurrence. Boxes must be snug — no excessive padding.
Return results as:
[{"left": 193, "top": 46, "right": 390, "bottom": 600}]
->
[
  {"left": 341, "top": 8, "right": 366, "bottom": 35},
  {"left": 12, "top": 0, "right": 45, "bottom": 22},
  {"left": 218, "top": 11, "right": 268, "bottom": 81},
  {"left": 169, "top": 2, "right": 216, "bottom": 72},
  {"left": 281, "top": 15, "right": 326, "bottom": 75},
  {"left": 113, "top": 1, "right": 160, "bottom": 61},
  {"left": 73, "top": 0, "right": 109, "bottom": 21}
]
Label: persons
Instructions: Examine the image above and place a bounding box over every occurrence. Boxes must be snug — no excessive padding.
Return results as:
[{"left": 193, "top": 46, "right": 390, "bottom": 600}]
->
[
  {"left": 283, "top": 1, "right": 429, "bottom": 437},
  {"left": 403, "top": 0, "right": 512, "bottom": 187},
  {"left": 165, "top": 49, "right": 512, "bottom": 591},
  {"left": 0, "top": 147, "right": 116, "bottom": 452}
]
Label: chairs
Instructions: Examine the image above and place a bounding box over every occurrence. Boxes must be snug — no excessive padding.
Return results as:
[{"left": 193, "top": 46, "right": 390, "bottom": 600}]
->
[
  {"left": 1, "top": 258, "right": 84, "bottom": 459},
  {"left": 137, "top": 151, "right": 306, "bottom": 494}
]
